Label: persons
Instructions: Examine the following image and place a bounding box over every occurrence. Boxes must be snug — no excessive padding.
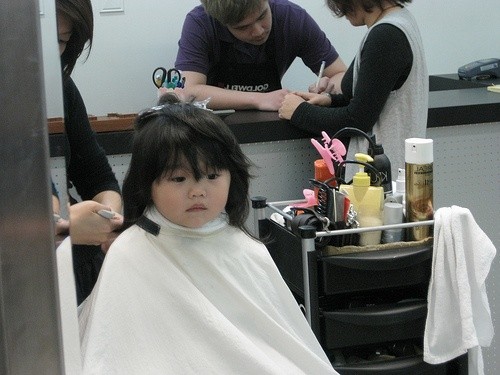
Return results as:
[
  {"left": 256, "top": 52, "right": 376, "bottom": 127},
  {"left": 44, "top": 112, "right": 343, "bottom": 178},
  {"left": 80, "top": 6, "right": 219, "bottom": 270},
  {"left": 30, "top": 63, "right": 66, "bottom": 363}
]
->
[
  {"left": 75, "top": 92, "right": 339, "bottom": 375},
  {"left": 55, "top": 0, "right": 125, "bottom": 306},
  {"left": 51, "top": 177, "right": 70, "bottom": 246},
  {"left": 175, "top": 0, "right": 348, "bottom": 111},
  {"left": 279, "top": 0, "right": 428, "bottom": 187}
]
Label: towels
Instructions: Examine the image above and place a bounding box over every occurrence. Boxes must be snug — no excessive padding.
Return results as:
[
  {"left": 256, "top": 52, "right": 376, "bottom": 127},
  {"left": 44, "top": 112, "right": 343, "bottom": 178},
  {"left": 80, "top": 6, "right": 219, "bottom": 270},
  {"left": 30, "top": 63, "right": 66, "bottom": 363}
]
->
[{"left": 422, "top": 205, "right": 497, "bottom": 365}]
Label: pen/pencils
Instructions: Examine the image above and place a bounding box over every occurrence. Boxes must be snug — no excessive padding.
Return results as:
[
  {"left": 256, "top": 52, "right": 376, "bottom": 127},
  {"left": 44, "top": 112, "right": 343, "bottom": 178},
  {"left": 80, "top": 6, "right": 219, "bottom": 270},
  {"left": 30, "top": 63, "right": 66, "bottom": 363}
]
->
[
  {"left": 152, "top": 72, "right": 185, "bottom": 102},
  {"left": 311, "top": 61, "right": 325, "bottom": 87}
]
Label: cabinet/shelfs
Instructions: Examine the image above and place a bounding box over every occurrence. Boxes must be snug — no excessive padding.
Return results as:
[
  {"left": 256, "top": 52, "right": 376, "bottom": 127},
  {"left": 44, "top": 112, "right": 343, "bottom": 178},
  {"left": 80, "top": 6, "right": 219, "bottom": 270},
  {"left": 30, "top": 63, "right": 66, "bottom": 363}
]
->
[{"left": 250, "top": 196, "right": 471, "bottom": 375}]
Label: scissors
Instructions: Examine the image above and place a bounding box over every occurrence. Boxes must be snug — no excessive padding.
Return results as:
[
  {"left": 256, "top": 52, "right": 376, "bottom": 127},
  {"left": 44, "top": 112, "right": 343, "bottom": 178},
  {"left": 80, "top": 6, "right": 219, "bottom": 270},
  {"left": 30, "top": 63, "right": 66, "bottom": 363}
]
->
[{"left": 152, "top": 68, "right": 181, "bottom": 89}]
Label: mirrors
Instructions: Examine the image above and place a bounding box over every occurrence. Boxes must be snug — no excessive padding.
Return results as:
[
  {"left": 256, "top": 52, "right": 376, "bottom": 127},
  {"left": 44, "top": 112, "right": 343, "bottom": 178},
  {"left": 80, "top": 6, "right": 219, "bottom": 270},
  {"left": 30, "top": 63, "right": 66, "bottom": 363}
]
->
[{"left": 40, "top": 0, "right": 500, "bottom": 375}]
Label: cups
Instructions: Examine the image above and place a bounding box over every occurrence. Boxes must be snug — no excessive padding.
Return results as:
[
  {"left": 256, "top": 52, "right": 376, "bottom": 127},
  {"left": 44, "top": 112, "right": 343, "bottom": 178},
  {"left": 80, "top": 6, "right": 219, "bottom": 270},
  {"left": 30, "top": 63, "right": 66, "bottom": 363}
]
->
[{"left": 157, "top": 87, "right": 183, "bottom": 100}]
